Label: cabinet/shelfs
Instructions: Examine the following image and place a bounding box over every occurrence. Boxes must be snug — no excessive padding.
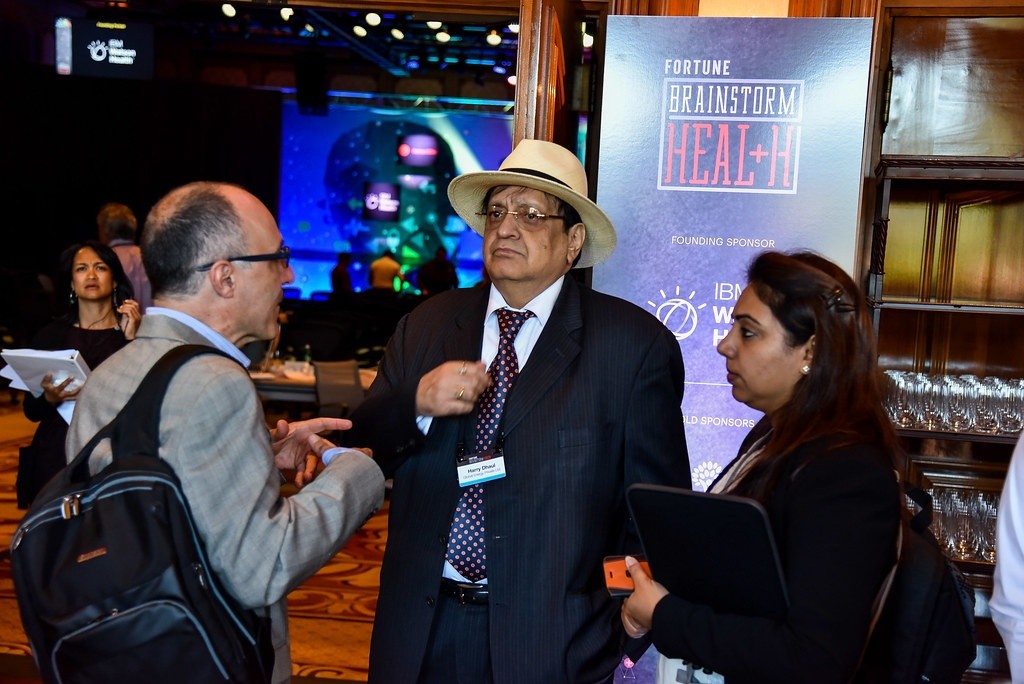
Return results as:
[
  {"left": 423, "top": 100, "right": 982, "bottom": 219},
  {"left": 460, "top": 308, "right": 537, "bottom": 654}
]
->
[{"left": 869, "top": 151, "right": 1024, "bottom": 680}]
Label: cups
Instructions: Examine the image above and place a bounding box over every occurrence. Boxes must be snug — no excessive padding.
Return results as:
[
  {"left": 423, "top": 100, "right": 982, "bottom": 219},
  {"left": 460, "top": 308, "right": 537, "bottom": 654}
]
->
[
  {"left": 905, "top": 489, "right": 998, "bottom": 563},
  {"left": 883, "top": 370, "right": 1024, "bottom": 434}
]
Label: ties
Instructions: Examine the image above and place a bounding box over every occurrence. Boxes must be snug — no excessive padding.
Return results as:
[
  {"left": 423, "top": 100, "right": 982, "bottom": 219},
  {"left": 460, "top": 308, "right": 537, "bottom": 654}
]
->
[{"left": 444, "top": 307, "right": 537, "bottom": 583}]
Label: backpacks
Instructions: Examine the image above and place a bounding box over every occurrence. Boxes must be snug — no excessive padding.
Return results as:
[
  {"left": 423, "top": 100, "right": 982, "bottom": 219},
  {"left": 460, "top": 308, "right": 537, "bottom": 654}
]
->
[
  {"left": 853, "top": 477, "right": 977, "bottom": 684},
  {"left": 9, "top": 340, "right": 276, "bottom": 684}
]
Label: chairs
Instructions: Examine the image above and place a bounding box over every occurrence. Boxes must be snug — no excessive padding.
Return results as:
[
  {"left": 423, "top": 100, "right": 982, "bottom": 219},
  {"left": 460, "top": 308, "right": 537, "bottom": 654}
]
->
[{"left": 311, "top": 361, "right": 367, "bottom": 419}]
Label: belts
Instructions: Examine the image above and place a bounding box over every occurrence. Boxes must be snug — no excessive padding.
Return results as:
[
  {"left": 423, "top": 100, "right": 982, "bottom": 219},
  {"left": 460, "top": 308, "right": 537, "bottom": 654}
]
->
[{"left": 434, "top": 577, "right": 493, "bottom": 609}]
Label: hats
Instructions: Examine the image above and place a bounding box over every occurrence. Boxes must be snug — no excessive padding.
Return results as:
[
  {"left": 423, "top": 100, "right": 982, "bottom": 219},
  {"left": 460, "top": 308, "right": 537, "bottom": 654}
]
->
[{"left": 448, "top": 139, "right": 618, "bottom": 269}]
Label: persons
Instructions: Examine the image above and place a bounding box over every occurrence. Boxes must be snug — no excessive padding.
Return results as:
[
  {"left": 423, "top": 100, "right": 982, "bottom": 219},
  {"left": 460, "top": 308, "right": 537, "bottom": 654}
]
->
[
  {"left": 323, "top": 120, "right": 459, "bottom": 289},
  {"left": 16, "top": 240, "right": 143, "bottom": 508},
  {"left": 622, "top": 251, "right": 900, "bottom": 684},
  {"left": 94, "top": 203, "right": 150, "bottom": 317},
  {"left": 63, "top": 181, "right": 386, "bottom": 684},
  {"left": 329, "top": 247, "right": 459, "bottom": 297},
  {"left": 350, "top": 140, "right": 692, "bottom": 684}
]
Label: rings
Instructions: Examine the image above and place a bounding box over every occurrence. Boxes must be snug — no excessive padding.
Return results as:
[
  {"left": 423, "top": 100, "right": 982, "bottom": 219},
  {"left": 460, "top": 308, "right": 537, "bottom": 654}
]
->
[
  {"left": 459, "top": 360, "right": 470, "bottom": 375},
  {"left": 456, "top": 386, "right": 464, "bottom": 400}
]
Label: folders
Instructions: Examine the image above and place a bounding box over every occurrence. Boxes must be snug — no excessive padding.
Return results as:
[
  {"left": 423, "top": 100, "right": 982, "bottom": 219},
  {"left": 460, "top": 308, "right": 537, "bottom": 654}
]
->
[{"left": 1, "top": 348, "right": 91, "bottom": 399}]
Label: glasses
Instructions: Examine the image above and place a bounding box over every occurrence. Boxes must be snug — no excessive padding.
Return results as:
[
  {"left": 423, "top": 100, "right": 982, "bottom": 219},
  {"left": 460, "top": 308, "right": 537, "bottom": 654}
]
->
[
  {"left": 474, "top": 204, "right": 563, "bottom": 233},
  {"left": 197, "top": 244, "right": 291, "bottom": 278}
]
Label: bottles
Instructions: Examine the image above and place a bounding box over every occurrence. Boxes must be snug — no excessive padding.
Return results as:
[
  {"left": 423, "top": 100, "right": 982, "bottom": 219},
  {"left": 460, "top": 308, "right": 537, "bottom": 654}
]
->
[{"left": 271, "top": 344, "right": 312, "bottom": 373}]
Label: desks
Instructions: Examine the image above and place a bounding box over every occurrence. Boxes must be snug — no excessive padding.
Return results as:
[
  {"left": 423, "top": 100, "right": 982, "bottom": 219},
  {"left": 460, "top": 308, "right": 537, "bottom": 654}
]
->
[{"left": 251, "top": 362, "right": 380, "bottom": 405}]
